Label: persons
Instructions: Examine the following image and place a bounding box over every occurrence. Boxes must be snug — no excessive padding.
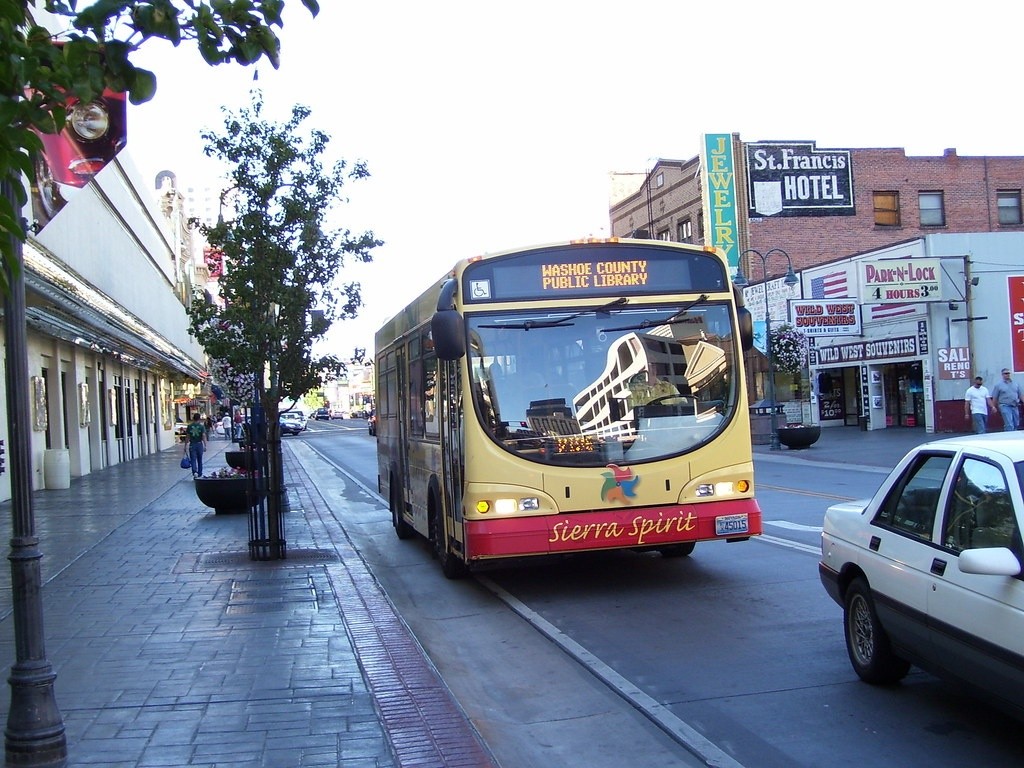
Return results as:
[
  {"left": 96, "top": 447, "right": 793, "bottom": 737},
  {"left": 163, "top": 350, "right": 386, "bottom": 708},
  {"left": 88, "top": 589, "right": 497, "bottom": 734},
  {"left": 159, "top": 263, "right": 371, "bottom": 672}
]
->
[
  {"left": 624, "top": 359, "right": 688, "bottom": 407},
  {"left": 234, "top": 411, "right": 242, "bottom": 439},
  {"left": 222, "top": 412, "right": 231, "bottom": 440},
  {"left": 185, "top": 413, "right": 207, "bottom": 479},
  {"left": 204, "top": 413, "right": 222, "bottom": 441},
  {"left": 176, "top": 415, "right": 183, "bottom": 423},
  {"left": 991, "top": 368, "right": 1024, "bottom": 431},
  {"left": 964, "top": 377, "right": 998, "bottom": 434}
]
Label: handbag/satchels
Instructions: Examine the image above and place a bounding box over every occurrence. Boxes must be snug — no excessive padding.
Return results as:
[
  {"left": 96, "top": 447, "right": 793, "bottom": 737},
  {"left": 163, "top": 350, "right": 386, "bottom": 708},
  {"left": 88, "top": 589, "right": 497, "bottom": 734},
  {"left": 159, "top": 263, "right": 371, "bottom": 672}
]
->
[{"left": 180, "top": 451, "right": 192, "bottom": 469}]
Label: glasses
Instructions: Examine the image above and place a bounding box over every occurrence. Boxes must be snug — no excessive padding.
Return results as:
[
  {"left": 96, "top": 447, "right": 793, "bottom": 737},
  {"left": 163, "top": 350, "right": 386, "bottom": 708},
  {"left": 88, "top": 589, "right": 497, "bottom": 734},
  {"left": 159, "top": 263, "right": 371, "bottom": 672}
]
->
[
  {"left": 978, "top": 383, "right": 982, "bottom": 386},
  {"left": 1002, "top": 372, "right": 1010, "bottom": 375}
]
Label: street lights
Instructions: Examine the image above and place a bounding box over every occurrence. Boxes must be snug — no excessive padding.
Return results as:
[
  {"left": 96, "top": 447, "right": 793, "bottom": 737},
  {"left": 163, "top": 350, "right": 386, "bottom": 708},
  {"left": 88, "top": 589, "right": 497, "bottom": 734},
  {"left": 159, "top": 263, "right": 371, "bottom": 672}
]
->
[
  {"left": 731, "top": 248, "right": 799, "bottom": 453},
  {"left": 217, "top": 181, "right": 326, "bottom": 514}
]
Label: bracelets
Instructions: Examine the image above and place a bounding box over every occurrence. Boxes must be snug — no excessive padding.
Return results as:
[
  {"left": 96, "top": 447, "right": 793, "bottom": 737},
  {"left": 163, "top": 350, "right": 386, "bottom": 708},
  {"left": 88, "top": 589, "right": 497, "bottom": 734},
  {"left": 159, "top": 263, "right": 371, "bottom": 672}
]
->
[{"left": 993, "top": 407, "right": 995, "bottom": 408}]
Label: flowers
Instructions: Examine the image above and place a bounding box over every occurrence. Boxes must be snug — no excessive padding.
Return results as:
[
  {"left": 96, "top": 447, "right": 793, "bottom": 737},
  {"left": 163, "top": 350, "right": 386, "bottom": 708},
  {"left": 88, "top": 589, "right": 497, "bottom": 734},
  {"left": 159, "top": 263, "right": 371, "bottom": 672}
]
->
[
  {"left": 235, "top": 443, "right": 264, "bottom": 452},
  {"left": 194, "top": 466, "right": 260, "bottom": 478},
  {"left": 778, "top": 423, "right": 816, "bottom": 428}
]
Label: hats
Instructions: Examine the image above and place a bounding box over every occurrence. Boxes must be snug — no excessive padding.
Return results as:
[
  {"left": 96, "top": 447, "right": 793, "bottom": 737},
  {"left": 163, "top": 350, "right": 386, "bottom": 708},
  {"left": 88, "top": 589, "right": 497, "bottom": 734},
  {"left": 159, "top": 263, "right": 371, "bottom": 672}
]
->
[{"left": 225, "top": 412, "right": 229, "bottom": 415}]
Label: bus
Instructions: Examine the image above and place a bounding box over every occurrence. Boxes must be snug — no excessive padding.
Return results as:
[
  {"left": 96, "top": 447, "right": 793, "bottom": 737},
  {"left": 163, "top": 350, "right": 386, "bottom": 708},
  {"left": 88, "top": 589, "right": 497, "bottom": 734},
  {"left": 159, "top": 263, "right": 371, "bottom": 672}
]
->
[{"left": 369, "top": 236, "right": 765, "bottom": 581}]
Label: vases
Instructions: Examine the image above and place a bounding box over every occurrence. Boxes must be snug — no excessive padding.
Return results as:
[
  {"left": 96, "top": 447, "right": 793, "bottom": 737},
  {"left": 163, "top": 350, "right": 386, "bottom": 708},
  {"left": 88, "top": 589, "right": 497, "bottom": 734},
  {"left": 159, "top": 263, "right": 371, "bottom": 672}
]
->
[
  {"left": 777, "top": 426, "right": 820, "bottom": 449},
  {"left": 225, "top": 451, "right": 268, "bottom": 468},
  {"left": 194, "top": 478, "right": 270, "bottom": 515}
]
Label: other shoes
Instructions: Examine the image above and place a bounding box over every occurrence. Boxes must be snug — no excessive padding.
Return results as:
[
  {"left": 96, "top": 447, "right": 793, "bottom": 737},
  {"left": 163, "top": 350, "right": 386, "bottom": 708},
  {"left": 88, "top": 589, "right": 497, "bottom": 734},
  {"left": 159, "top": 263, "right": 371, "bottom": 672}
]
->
[{"left": 192, "top": 473, "right": 198, "bottom": 480}]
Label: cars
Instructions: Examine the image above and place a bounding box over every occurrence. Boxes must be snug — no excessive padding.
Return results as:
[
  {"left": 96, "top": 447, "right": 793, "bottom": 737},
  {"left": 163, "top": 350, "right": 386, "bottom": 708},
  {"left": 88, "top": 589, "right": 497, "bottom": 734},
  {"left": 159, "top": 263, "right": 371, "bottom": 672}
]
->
[
  {"left": 215, "top": 414, "right": 252, "bottom": 436},
  {"left": 818, "top": 429, "right": 1024, "bottom": 727},
  {"left": 278, "top": 409, "right": 308, "bottom": 437},
  {"left": 367, "top": 409, "right": 376, "bottom": 436},
  {"left": 309, "top": 407, "right": 344, "bottom": 420}
]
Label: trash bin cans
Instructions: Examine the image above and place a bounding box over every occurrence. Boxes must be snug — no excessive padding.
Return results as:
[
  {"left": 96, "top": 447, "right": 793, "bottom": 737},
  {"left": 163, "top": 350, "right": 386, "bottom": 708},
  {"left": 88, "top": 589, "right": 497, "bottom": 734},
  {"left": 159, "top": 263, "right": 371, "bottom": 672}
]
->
[{"left": 749, "top": 399, "right": 787, "bottom": 445}]
